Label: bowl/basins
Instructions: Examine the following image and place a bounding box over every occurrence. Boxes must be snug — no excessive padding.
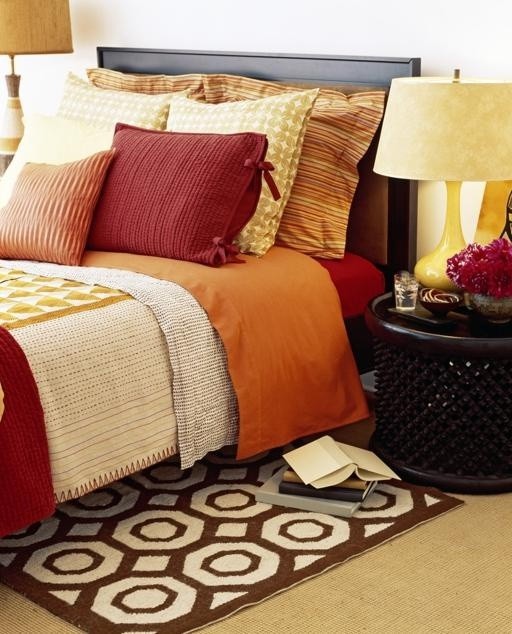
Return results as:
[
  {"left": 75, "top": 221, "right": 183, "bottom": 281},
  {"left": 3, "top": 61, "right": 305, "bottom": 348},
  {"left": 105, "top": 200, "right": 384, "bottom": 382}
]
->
[{"left": 417, "top": 293, "right": 465, "bottom": 316}]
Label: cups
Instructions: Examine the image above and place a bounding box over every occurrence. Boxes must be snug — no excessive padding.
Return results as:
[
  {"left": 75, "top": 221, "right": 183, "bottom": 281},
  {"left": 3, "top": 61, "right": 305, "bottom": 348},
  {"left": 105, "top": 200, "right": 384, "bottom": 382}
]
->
[{"left": 394, "top": 274, "right": 417, "bottom": 311}]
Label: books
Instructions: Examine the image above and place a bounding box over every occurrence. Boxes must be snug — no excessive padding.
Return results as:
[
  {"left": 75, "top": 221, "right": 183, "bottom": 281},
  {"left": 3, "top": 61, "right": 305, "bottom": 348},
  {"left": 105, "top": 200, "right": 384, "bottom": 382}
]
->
[{"left": 255, "top": 434, "right": 403, "bottom": 519}]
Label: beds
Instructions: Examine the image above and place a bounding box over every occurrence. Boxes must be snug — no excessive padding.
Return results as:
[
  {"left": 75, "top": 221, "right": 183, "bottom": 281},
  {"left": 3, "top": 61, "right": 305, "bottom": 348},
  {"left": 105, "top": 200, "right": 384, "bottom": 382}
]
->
[{"left": 0, "top": 44, "right": 421, "bottom": 539}]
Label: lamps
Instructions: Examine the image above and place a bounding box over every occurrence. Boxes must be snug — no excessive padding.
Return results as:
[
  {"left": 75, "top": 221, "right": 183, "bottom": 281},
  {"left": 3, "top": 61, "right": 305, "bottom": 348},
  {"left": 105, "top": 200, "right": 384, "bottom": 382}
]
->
[
  {"left": 370, "top": 66, "right": 512, "bottom": 294},
  {"left": 0, "top": 0, "right": 73, "bottom": 180}
]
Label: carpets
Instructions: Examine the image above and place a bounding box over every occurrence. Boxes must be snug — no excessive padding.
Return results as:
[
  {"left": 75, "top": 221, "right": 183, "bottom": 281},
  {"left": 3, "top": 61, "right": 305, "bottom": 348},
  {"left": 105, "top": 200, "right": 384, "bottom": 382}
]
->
[{"left": 0, "top": 437, "right": 467, "bottom": 633}]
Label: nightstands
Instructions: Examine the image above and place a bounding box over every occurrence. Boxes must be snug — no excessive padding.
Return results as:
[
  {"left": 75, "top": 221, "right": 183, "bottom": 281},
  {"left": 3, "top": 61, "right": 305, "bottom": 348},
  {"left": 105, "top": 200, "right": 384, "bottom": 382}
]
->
[{"left": 360, "top": 288, "right": 511, "bottom": 497}]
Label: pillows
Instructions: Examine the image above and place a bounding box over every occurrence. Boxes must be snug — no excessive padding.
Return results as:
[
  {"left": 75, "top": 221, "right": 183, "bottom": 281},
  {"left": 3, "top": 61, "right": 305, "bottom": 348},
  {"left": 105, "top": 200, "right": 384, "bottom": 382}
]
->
[
  {"left": 85, "top": 121, "right": 282, "bottom": 267},
  {"left": 200, "top": 71, "right": 387, "bottom": 262},
  {"left": 1, "top": 146, "right": 117, "bottom": 267},
  {"left": 84, "top": 64, "right": 209, "bottom": 106},
  {"left": 166, "top": 85, "right": 322, "bottom": 260},
  {"left": 24, "top": 69, "right": 193, "bottom": 163},
  {"left": 0, "top": 113, "right": 114, "bottom": 210}
]
